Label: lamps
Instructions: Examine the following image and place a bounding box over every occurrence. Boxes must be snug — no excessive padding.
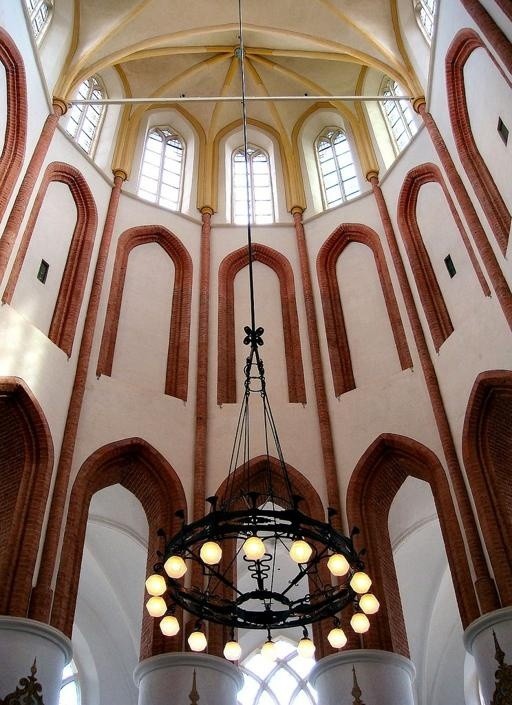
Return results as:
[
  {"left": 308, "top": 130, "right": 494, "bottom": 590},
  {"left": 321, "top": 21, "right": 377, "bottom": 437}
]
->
[{"left": 144, "top": 0, "right": 381, "bottom": 661}]
